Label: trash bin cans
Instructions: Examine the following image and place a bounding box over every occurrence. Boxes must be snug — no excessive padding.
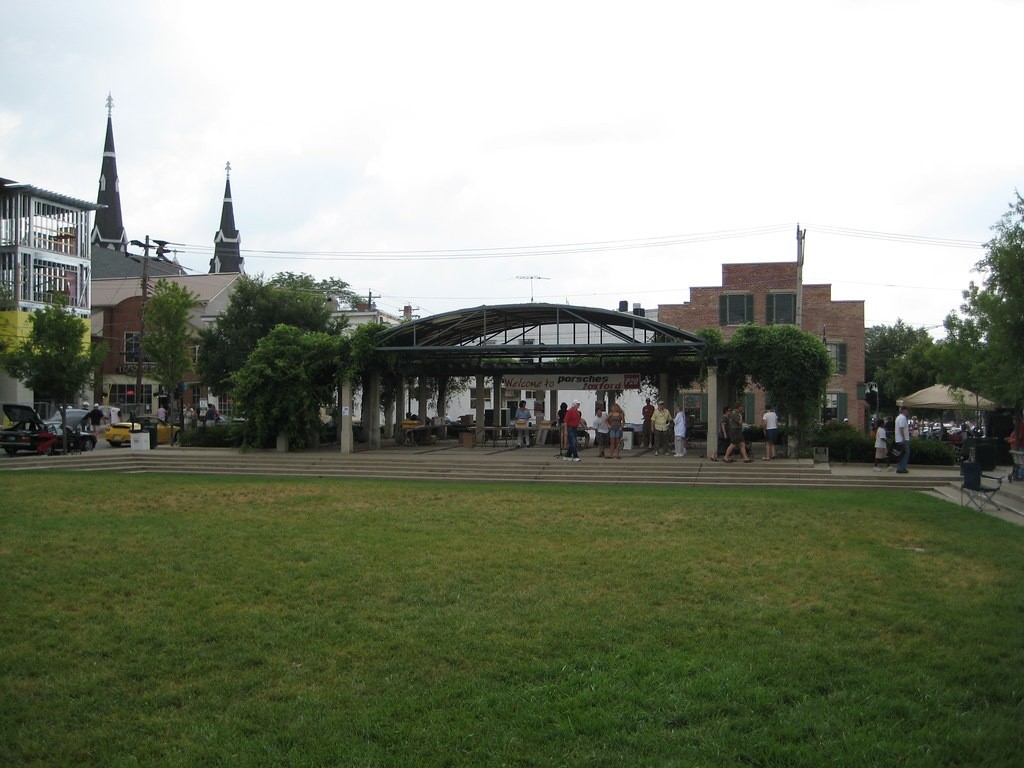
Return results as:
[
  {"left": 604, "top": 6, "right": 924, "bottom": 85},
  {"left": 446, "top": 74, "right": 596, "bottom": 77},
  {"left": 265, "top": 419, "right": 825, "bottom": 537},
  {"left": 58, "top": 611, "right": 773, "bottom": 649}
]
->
[
  {"left": 128, "top": 430, "right": 150, "bottom": 451},
  {"left": 622, "top": 428, "right": 634, "bottom": 450},
  {"left": 139, "top": 418, "right": 159, "bottom": 449}
]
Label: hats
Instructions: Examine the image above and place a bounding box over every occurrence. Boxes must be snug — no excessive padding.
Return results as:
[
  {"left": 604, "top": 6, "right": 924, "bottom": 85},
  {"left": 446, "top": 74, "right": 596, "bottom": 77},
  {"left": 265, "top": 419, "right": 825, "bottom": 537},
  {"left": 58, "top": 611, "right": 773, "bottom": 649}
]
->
[
  {"left": 83, "top": 402, "right": 89, "bottom": 405},
  {"left": 94, "top": 403, "right": 99, "bottom": 407},
  {"left": 646, "top": 397, "right": 650, "bottom": 400},
  {"left": 659, "top": 400, "right": 664, "bottom": 405},
  {"left": 573, "top": 399, "right": 580, "bottom": 404}
]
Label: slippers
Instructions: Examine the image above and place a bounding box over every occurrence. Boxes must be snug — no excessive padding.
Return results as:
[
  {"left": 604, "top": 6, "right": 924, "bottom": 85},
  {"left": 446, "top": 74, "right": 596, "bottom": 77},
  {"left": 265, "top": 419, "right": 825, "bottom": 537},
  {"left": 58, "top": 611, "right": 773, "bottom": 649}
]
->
[
  {"left": 723, "top": 457, "right": 731, "bottom": 463},
  {"left": 744, "top": 459, "right": 754, "bottom": 463},
  {"left": 730, "top": 458, "right": 737, "bottom": 462},
  {"left": 711, "top": 457, "right": 717, "bottom": 462}
]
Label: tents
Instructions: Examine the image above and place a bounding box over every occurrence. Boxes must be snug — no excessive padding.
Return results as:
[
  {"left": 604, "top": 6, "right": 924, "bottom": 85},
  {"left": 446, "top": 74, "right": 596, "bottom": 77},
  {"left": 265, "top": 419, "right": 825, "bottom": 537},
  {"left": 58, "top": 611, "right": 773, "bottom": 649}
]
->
[{"left": 896, "top": 383, "right": 995, "bottom": 431}]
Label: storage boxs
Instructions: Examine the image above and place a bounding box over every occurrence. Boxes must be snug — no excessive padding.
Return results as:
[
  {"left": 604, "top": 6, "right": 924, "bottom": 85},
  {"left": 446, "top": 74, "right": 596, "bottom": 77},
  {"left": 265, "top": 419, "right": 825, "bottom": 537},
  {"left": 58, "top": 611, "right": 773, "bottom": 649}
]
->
[{"left": 402, "top": 420, "right": 419, "bottom": 428}]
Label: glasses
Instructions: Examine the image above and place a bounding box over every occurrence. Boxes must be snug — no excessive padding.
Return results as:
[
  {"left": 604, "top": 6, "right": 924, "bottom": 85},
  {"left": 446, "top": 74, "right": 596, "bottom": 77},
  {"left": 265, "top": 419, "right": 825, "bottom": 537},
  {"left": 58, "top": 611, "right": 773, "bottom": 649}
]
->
[{"left": 726, "top": 410, "right": 731, "bottom": 412}]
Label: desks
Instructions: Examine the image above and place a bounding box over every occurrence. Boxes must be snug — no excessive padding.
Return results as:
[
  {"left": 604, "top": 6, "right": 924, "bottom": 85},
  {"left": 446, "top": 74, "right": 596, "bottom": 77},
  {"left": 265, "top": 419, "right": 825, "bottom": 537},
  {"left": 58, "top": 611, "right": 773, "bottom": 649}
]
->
[
  {"left": 406, "top": 425, "right": 461, "bottom": 446},
  {"left": 468, "top": 426, "right": 595, "bottom": 448}
]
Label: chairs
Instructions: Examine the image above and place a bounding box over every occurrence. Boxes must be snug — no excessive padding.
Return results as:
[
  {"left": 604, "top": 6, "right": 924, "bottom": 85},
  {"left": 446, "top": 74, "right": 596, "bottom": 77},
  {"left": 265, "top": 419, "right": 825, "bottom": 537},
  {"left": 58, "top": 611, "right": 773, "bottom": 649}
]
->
[{"left": 960, "top": 460, "right": 1005, "bottom": 509}]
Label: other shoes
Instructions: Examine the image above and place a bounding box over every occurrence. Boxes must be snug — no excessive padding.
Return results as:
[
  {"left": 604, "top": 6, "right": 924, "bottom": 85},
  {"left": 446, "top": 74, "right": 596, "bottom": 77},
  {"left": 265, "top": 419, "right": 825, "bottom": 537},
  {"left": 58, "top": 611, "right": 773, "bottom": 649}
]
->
[
  {"left": 563, "top": 456, "right": 573, "bottom": 461},
  {"left": 517, "top": 445, "right": 520, "bottom": 448},
  {"left": 574, "top": 458, "right": 581, "bottom": 462},
  {"left": 655, "top": 451, "right": 658, "bottom": 456},
  {"left": 673, "top": 454, "right": 683, "bottom": 458},
  {"left": 604, "top": 456, "right": 613, "bottom": 459},
  {"left": 888, "top": 466, "right": 896, "bottom": 472},
  {"left": 665, "top": 452, "right": 670, "bottom": 456},
  {"left": 616, "top": 456, "right": 621, "bottom": 459},
  {"left": 897, "top": 469, "right": 908, "bottom": 473},
  {"left": 873, "top": 466, "right": 882, "bottom": 472},
  {"left": 527, "top": 445, "right": 531, "bottom": 448},
  {"left": 762, "top": 457, "right": 769, "bottom": 461},
  {"left": 598, "top": 454, "right": 604, "bottom": 457}
]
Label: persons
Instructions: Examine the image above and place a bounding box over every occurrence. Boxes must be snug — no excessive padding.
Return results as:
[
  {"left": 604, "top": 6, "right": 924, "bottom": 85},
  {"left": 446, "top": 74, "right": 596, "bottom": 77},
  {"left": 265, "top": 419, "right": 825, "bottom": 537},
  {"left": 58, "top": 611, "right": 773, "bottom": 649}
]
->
[
  {"left": 2, "top": 414, "right": 13, "bottom": 428},
  {"left": 639, "top": 397, "right": 687, "bottom": 458},
  {"left": 593, "top": 404, "right": 625, "bottom": 459},
  {"left": 514, "top": 400, "right": 531, "bottom": 449},
  {"left": 556, "top": 402, "right": 590, "bottom": 450},
  {"left": 760, "top": 404, "right": 778, "bottom": 461},
  {"left": 956, "top": 425, "right": 973, "bottom": 461},
  {"left": 871, "top": 417, "right": 894, "bottom": 437},
  {"left": 109, "top": 402, "right": 122, "bottom": 424},
  {"left": 205, "top": 403, "right": 219, "bottom": 427},
  {"left": 400, "top": 412, "right": 450, "bottom": 444},
  {"left": 80, "top": 402, "right": 105, "bottom": 436},
  {"left": 710, "top": 404, "right": 754, "bottom": 464},
  {"left": 1008, "top": 419, "right": 1024, "bottom": 481},
  {"left": 563, "top": 403, "right": 581, "bottom": 462},
  {"left": 156, "top": 402, "right": 198, "bottom": 431},
  {"left": 873, "top": 419, "right": 894, "bottom": 471},
  {"left": 894, "top": 406, "right": 910, "bottom": 473}
]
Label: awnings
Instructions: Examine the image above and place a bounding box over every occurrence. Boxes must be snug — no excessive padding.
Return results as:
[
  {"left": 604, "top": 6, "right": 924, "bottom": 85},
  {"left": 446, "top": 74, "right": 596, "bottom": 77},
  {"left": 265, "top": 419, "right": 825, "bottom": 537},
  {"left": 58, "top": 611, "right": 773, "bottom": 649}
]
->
[{"left": 103, "top": 374, "right": 163, "bottom": 398}]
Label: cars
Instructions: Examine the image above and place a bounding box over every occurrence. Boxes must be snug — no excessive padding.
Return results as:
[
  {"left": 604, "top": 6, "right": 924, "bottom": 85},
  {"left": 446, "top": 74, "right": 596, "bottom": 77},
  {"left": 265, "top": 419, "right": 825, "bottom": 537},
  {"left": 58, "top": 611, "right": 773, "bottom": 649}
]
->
[
  {"left": 103, "top": 416, "right": 181, "bottom": 448},
  {"left": 0, "top": 403, "right": 98, "bottom": 457}
]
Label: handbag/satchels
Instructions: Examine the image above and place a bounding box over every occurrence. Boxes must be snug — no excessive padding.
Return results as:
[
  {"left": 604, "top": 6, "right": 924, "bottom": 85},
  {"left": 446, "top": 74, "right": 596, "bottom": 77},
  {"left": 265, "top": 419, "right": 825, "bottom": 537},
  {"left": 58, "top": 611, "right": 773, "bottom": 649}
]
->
[{"left": 891, "top": 443, "right": 904, "bottom": 456}]
